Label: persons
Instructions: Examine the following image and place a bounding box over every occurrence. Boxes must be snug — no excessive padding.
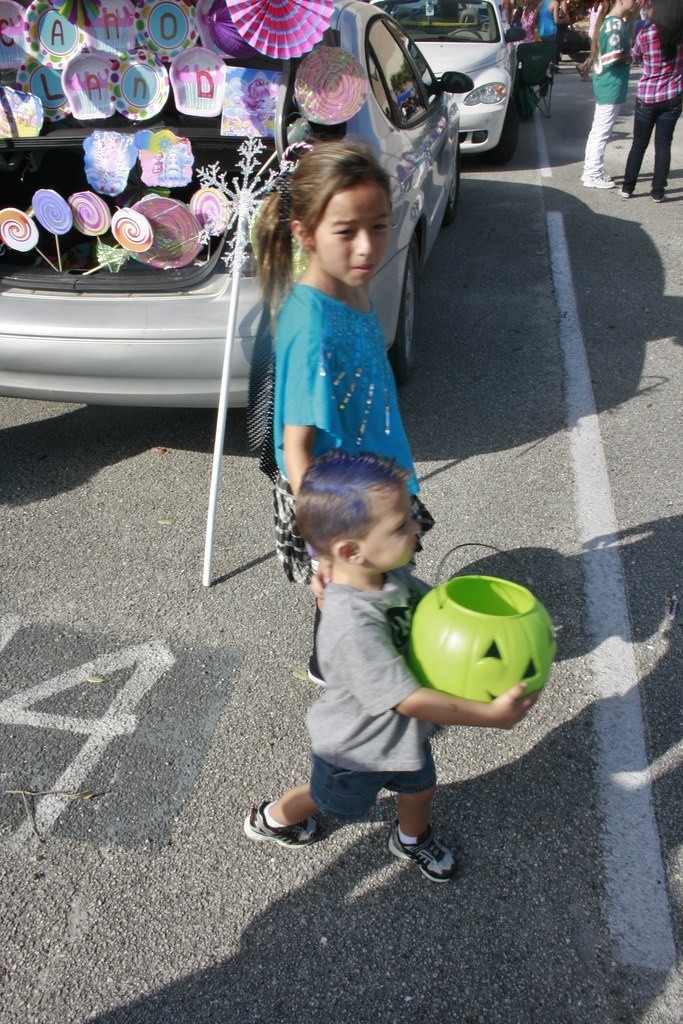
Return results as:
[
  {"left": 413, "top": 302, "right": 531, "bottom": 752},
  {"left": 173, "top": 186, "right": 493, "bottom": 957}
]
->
[
  {"left": 504, "top": 0, "right": 611, "bottom": 80},
  {"left": 249, "top": 144, "right": 435, "bottom": 688},
  {"left": 244, "top": 451, "right": 545, "bottom": 887},
  {"left": 385, "top": 96, "right": 418, "bottom": 125},
  {"left": 580, "top": 1, "right": 638, "bottom": 190},
  {"left": 614, "top": 0, "right": 683, "bottom": 203}
]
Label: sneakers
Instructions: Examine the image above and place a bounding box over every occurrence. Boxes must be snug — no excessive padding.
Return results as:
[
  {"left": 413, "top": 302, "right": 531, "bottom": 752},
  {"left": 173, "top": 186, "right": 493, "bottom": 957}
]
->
[
  {"left": 240, "top": 803, "right": 321, "bottom": 848},
  {"left": 387, "top": 818, "right": 457, "bottom": 884},
  {"left": 581, "top": 173, "right": 614, "bottom": 188}
]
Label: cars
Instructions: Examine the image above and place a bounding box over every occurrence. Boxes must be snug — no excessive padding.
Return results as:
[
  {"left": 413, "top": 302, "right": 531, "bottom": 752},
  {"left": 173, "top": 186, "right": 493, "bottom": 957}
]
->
[
  {"left": 369, "top": 0, "right": 528, "bottom": 169},
  {"left": 2, "top": 10, "right": 476, "bottom": 412}
]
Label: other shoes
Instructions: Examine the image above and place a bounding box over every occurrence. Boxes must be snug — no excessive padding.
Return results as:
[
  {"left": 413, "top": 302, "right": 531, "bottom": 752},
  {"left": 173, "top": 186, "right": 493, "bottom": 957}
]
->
[{"left": 618, "top": 185, "right": 668, "bottom": 203}]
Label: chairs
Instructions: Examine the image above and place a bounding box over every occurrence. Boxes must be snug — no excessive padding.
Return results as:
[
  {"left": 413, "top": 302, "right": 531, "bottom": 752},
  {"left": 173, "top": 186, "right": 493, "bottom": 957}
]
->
[
  {"left": 449, "top": 8, "right": 489, "bottom": 42},
  {"left": 512, "top": 41, "right": 558, "bottom": 117}
]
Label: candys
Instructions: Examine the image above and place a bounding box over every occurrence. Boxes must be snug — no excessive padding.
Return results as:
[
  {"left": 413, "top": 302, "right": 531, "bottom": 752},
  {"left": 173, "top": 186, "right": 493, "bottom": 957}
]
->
[
  {"left": 0, "top": 185, "right": 231, "bottom": 275},
  {"left": 241, "top": 46, "right": 366, "bottom": 194}
]
